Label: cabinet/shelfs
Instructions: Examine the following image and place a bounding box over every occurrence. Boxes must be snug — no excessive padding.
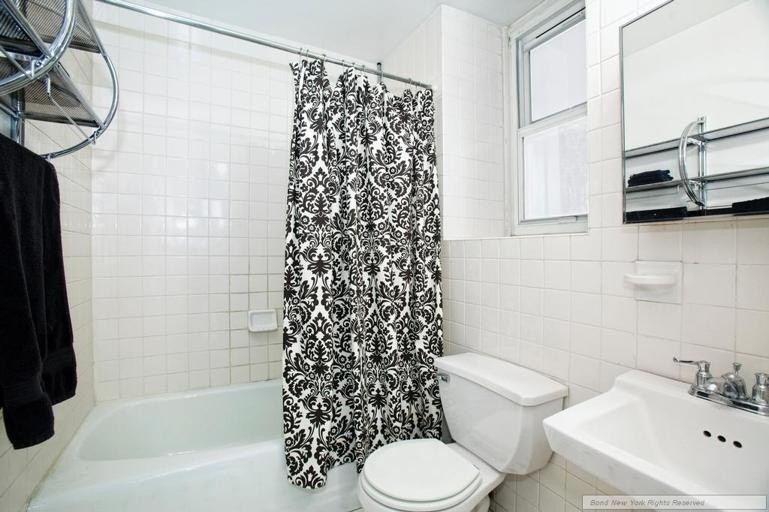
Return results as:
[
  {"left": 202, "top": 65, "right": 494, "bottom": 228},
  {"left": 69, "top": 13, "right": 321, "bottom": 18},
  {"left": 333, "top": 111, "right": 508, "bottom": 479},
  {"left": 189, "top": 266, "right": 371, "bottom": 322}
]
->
[
  {"left": 624, "top": 114, "right": 769, "bottom": 211},
  {"left": 0, "top": 0, "right": 119, "bottom": 160}
]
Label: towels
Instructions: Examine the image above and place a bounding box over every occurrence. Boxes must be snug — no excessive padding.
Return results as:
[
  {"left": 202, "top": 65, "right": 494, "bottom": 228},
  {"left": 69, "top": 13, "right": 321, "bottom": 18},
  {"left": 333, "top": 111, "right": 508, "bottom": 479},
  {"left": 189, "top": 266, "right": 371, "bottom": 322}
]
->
[
  {"left": 0, "top": 134, "right": 77, "bottom": 450},
  {"left": 627, "top": 170, "right": 675, "bottom": 186}
]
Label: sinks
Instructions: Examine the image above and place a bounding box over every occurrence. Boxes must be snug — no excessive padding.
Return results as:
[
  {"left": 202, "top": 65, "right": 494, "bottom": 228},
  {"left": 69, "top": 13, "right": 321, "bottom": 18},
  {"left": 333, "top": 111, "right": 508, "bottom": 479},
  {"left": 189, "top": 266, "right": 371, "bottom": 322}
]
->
[{"left": 543, "top": 373, "right": 768, "bottom": 511}]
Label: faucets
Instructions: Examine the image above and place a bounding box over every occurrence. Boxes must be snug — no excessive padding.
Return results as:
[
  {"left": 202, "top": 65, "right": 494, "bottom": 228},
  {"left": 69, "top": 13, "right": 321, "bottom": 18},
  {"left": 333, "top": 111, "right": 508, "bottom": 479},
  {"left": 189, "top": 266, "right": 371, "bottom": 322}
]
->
[{"left": 696, "top": 362, "right": 750, "bottom": 406}]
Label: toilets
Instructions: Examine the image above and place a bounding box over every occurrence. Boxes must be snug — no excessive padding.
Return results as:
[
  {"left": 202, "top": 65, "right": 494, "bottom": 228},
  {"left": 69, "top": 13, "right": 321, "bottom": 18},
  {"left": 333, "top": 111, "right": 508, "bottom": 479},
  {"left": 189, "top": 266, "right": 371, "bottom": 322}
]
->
[{"left": 355, "top": 351, "right": 568, "bottom": 511}]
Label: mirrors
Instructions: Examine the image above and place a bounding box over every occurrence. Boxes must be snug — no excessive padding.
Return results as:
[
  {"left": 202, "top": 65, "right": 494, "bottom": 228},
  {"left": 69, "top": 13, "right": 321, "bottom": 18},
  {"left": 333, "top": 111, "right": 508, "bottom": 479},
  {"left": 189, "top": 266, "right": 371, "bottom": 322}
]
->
[{"left": 618, "top": 0, "right": 769, "bottom": 223}]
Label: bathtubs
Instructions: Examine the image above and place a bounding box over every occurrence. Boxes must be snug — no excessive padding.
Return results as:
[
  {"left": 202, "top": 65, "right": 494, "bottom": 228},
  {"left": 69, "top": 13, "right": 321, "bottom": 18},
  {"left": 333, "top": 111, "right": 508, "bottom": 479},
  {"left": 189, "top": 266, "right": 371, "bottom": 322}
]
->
[{"left": 24, "top": 377, "right": 354, "bottom": 512}]
